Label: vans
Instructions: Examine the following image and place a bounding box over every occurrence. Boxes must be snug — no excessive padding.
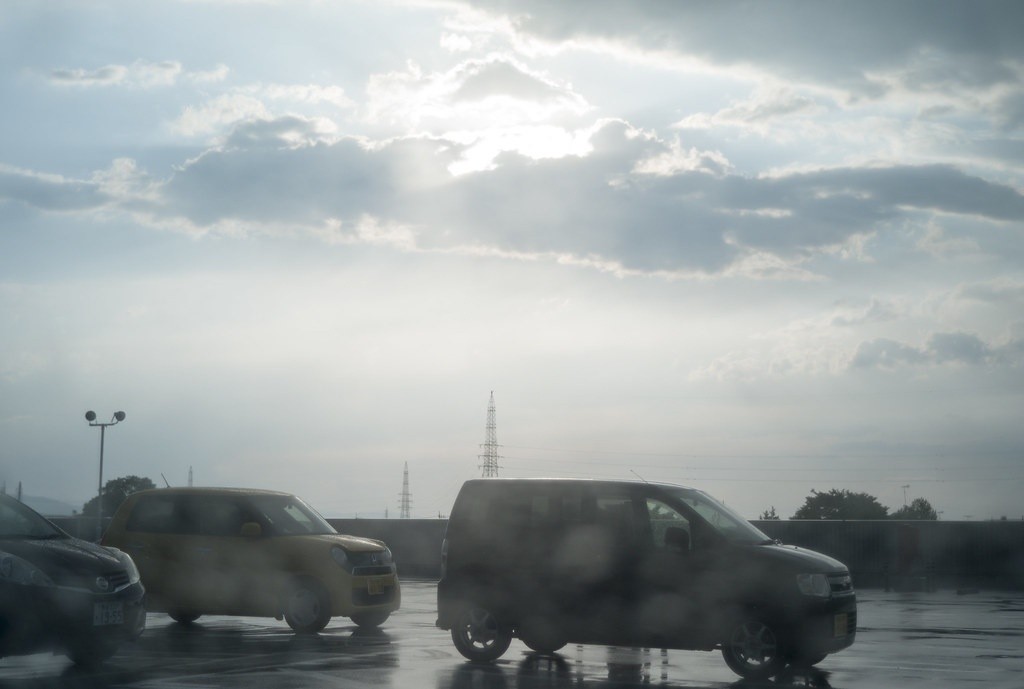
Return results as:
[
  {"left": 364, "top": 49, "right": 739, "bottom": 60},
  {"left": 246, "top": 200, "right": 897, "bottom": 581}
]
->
[
  {"left": 436, "top": 479, "right": 858, "bottom": 679},
  {"left": 99, "top": 488, "right": 401, "bottom": 636},
  {"left": 0, "top": 491, "right": 148, "bottom": 665}
]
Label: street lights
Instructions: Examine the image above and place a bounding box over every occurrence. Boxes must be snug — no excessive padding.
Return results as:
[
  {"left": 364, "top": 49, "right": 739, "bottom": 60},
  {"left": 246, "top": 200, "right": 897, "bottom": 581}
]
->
[
  {"left": 86, "top": 409, "right": 124, "bottom": 489},
  {"left": 902, "top": 485, "right": 909, "bottom": 504}
]
